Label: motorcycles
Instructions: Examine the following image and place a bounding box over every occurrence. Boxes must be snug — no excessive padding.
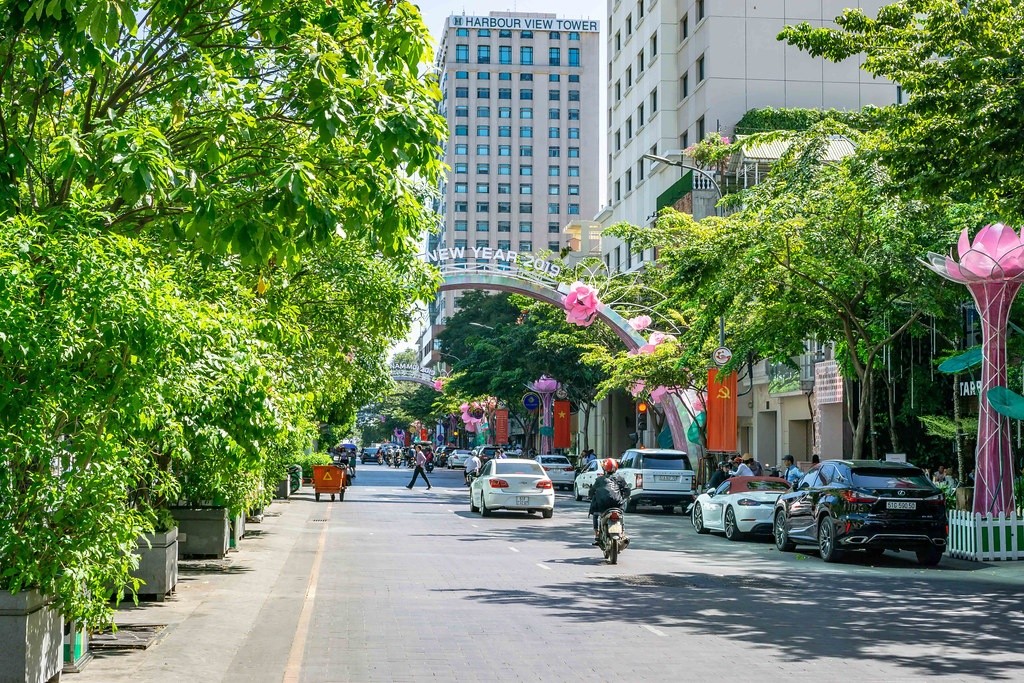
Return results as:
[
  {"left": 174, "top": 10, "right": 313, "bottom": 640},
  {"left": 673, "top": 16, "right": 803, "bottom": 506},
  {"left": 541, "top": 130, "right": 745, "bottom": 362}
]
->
[
  {"left": 585, "top": 495, "right": 631, "bottom": 565},
  {"left": 375, "top": 444, "right": 435, "bottom": 473}
]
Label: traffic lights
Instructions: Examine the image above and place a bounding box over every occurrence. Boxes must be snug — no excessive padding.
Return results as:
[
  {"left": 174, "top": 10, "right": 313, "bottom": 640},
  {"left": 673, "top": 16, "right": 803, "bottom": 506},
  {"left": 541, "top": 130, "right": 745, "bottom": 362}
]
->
[
  {"left": 636, "top": 397, "right": 648, "bottom": 431},
  {"left": 640, "top": 446, "right": 645, "bottom": 449},
  {"left": 453, "top": 431, "right": 458, "bottom": 438}
]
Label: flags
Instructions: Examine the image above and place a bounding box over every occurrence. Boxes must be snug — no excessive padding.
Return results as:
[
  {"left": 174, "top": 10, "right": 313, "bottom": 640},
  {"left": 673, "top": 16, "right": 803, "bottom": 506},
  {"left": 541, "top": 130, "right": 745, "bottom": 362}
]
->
[
  {"left": 496, "top": 410, "right": 508, "bottom": 444},
  {"left": 554, "top": 401, "right": 570, "bottom": 448},
  {"left": 707, "top": 368, "right": 737, "bottom": 451}
]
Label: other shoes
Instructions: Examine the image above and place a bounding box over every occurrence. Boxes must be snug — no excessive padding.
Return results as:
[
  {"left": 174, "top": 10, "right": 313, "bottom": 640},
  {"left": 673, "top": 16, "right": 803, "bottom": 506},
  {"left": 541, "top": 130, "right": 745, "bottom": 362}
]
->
[
  {"left": 406, "top": 486, "right": 411, "bottom": 489},
  {"left": 466, "top": 482, "right": 469, "bottom": 484},
  {"left": 595, "top": 535, "right": 601, "bottom": 541},
  {"left": 426, "top": 485, "right": 432, "bottom": 490}
]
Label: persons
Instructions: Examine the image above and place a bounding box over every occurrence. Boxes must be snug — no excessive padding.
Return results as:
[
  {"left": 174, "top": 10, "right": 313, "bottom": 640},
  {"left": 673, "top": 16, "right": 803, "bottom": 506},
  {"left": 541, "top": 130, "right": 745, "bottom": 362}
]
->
[
  {"left": 589, "top": 458, "right": 630, "bottom": 546},
  {"left": 809, "top": 454, "right": 820, "bottom": 469},
  {"left": 702, "top": 457, "right": 762, "bottom": 493},
  {"left": 464, "top": 450, "right": 481, "bottom": 485},
  {"left": 932, "top": 465, "right": 975, "bottom": 492},
  {"left": 781, "top": 455, "right": 803, "bottom": 484},
  {"left": 375, "top": 446, "right": 434, "bottom": 469},
  {"left": 494, "top": 448, "right": 507, "bottom": 459},
  {"left": 325, "top": 447, "right": 356, "bottom": 478},
  {"left": 405, "top": 445, "right": 433, "bottom": 490}
]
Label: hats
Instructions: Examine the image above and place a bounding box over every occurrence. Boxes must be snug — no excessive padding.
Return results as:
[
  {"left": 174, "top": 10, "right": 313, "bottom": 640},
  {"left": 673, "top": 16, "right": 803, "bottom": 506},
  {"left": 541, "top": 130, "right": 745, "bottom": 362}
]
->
[
  {"left": 782, "top": 455, "right": 794, "bottom": 461},
  {"left": 733, "top": 457, "right": 743, "bottom": 463}
]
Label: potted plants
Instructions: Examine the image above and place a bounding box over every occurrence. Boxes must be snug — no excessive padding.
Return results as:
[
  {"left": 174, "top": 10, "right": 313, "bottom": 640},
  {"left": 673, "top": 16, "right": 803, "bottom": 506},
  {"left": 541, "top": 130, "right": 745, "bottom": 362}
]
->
[{"left": 0, "top": 183, "right": 323, "bottom": 683}]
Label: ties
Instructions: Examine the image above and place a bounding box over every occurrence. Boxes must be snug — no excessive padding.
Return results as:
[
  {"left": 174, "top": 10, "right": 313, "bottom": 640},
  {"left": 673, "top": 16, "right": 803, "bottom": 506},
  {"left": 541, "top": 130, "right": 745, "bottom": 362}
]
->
[{"left": 786, "top": 468, "right": 791, "bottom": 480}]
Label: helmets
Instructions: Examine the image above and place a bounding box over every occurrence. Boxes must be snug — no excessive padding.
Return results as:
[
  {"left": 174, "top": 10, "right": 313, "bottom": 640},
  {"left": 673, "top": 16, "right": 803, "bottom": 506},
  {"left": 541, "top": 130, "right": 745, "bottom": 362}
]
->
[
  {"left": 471, "top": 450, "right": 477, "bottom": 456},
  {"left": 601, "top": 458, "right": 618, "bottom": 473},
  {"left": 718, "top": 461, "right": 730, "bottom": 470}
]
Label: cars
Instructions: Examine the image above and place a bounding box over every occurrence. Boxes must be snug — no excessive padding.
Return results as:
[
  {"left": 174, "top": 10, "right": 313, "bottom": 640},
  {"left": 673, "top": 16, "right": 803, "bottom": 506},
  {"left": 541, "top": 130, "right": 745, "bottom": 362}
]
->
[
  {"left": 531, "top": 454, "right": 576, "bottom": 492},
  {"left": 447, "top": 448, "right": 472, "bottom": 470},
  {"left": 358, "top": 447, "right": 379, "bottom": 464},
  {"left": 467, "top": 458, "right": 554, "bottom": 518},
  {"left": 692, "top": 473, "right": 796, "bottom": 541},
  {"left": 572, "top": 457, "right": 625, "bottom": 501}
]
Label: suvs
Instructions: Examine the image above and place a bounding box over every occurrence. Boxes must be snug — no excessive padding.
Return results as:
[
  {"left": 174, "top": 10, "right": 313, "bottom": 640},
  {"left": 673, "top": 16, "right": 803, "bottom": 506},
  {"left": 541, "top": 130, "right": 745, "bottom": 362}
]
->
[
  {"left": 472, "top": 443, "right": 524, "bottom": 459},
  {"left": 770, "top": 458, "right": 951, "bottom": 569},
  {"left": 611, "top": 448, "right": 696, "bottom": 516},
  {"left": 433, "top": 446, "right": 459, "bottom": 468}
]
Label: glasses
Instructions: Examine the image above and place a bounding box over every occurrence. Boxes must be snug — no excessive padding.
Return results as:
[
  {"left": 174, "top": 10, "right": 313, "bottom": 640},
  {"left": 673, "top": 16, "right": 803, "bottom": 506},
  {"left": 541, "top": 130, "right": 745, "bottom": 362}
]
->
[{"left": 723, "top": 465, "right": 729, "bottom": 468}]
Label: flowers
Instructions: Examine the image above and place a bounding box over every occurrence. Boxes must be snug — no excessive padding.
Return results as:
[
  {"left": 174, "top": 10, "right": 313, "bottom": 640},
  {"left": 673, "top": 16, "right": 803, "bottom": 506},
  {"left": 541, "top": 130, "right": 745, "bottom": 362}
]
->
[
  {"left": 459, "top": 398, "right": 498, "bottom": 433},
  {"left": 393, "top": 427, "right": 405, "bottom": 442},
  {"left": 533, "top": 374, "right": 558, "bottom": 454},
  {"left": 433, "top": 379, "right": 443, "bottom": 392},
  {"left": 626, "top": 314, "right": 709, "bottom": 412},
  {"left": 945, "top": 222, "right": 1024, "bottom": 515},
  {"left": 560, "top": 279, "right": 602, "bottom": 328}
]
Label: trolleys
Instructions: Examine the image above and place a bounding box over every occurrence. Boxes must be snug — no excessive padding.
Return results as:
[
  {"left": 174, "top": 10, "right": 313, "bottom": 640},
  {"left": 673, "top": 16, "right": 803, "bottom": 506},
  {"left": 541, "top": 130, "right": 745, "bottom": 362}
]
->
[{"left": 311, "top": 461, "right": 348, "bottom": 502}]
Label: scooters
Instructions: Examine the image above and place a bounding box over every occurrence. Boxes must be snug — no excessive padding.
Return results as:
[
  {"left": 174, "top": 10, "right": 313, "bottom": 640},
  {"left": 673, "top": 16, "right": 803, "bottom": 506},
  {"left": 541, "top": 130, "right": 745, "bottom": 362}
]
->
[{"left": 289, "top": 466, "right": 302, "bottom": 493}]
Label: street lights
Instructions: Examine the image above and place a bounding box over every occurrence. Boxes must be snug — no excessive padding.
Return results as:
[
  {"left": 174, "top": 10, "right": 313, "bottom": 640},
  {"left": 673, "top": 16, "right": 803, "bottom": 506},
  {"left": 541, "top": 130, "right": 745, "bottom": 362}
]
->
[{"left": 641, "top": 154, "right": 726, "bottom": 465}]
